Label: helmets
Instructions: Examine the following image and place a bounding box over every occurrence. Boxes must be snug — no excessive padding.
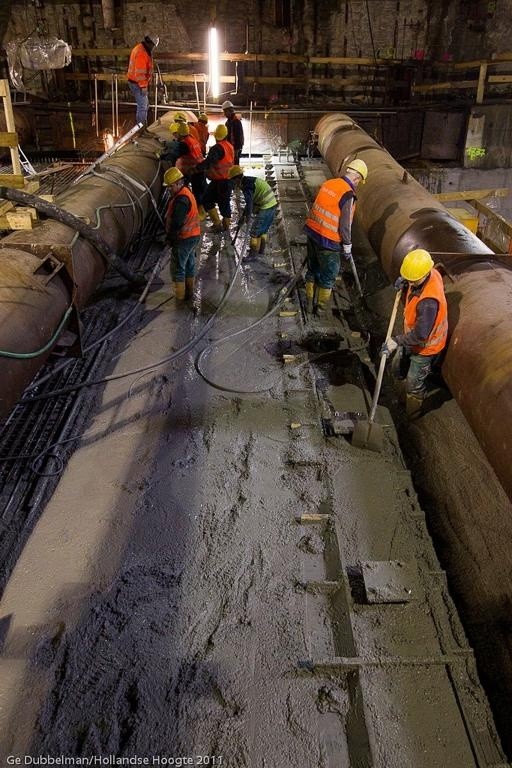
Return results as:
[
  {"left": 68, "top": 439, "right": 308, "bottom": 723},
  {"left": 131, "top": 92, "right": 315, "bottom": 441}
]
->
[
  {"left": 214, "top": 123, "right": 229, "bottom": 141},
  {"left": 163, "top": 166, "right": 185, "bottom": 186},
  {"left": 400, "top": 249, "right": 435, "bottom": 282},
  {"left": 224, "top": 164, "right": 244, "bottom": 178},
  {"left": 346, "top": 159, "right": 368, "bottom": 184},
  {"left": 148, "top": 33, "right": 159, "bottom": 47},
  {"left": 219, "top": 100, "right": 234, "bottom": 110},
  {"left": 162, "top": 100, "right": 209, "bottom": 135}
]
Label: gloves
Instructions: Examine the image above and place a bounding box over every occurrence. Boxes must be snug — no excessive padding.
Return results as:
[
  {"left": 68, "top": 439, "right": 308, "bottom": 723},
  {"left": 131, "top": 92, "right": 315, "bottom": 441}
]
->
[
  {"left": 379, "top": 336, "right": 399, "bottom": 358},
  {"left": 395, "top": 276, "right": 408, "bottom": 291},
  {"left": 240, "top": 215, "right": 252, "bottom": 226},
  {"left": 342, "top": 243, "right": 352, "bottom": 260},
  {"left": 165, "top": 232, "right": 178, "bottom": 246}
]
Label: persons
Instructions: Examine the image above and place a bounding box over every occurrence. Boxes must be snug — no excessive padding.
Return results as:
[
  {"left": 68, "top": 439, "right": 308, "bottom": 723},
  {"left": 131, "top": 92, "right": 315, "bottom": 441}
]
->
[
  {"left": 221, "top": 100, "right": 244, "bottom": 166},
  {"left": 380, "top": 247, "right": 450, "bottom": 415},
  {"left": 126, "top": 32, "right": 161, "bottom": 138},
  {"left": 302, "top": 158, "right": 368, "bottom": 317},
  {"left": 228, "top": 164, "right": 277, "bottom": 263},
  {"left": 196, "top": 124, "right": 236, "bottom": 234},
  {"left": 156, "top": 105, "right": 209, "bottom": 221},
  {"left": 162, "top": 167, "right": 200, "bottom": 290}
]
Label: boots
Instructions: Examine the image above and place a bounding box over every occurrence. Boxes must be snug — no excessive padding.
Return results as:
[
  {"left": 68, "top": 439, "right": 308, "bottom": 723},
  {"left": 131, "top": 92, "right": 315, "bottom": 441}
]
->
[
  {"left": 137, "top": 122, "right": 156, "bottom": 138},
  {"left": 392, "top": 378, "right": 424, "bottom": 421},
  {"left": 171, "top": 276, "right": 195, "bottom": 310},
  {"left": 205, "top": 207, "right": 230, "bottom": 233},
  {"left": 305, "top": 279, "right": 333, "bottom": 316},
  {"left": 242, "top": 233, "right": 268, "bottom": 262}
]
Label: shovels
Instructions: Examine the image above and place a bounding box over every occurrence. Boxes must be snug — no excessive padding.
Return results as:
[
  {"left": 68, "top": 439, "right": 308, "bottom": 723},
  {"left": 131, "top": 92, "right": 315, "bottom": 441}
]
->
[{"left": 351, "top": 283, "right": 406, "bottom": 453}]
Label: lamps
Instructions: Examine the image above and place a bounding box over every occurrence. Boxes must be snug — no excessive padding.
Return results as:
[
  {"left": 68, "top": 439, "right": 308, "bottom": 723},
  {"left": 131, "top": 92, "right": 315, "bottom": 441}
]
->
[{"left": 212, "top": 26, "right": 219, "bottom": 104}]
